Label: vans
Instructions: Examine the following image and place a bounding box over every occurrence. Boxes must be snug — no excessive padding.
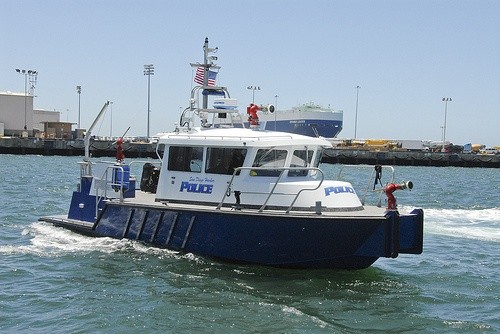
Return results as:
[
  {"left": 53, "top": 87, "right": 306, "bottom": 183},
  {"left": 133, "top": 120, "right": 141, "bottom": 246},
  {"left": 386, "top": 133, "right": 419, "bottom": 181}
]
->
[{"left": 484, "top": 147, "right": 498, "bottom": 154}]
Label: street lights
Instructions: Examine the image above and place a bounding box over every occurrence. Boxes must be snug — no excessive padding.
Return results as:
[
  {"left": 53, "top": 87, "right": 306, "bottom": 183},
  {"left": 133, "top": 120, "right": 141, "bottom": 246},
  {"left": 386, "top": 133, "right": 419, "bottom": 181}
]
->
[
  {"left": 247, "top": 86, "right": 260, "bottom": 103},
  {"left": 143, "top": 64, "right": 154, "bottom": 137},
  {"left": 354, "top": 85, "right": 360, "bottom": 138},
  {"left": 76, "top": 85, "right": 82, "bottom": 128},
  {"left": 109, "top": 102, "right": 114, "bottom": 138},
  {"left": 15, "top": 68, "right": 36, "bottom": 132},
  {"left": 441, "top": 97, "right": 453, "bottom": 153}
]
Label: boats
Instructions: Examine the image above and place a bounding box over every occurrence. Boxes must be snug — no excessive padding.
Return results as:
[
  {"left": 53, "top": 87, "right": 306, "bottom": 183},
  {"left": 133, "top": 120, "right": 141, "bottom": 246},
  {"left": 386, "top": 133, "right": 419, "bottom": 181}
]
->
[
  {"left": 206, "top": 93, "right": 344, "bottom": 138},
  {"left": 38, "top": 36, "right": 424, "bottom": 270}
]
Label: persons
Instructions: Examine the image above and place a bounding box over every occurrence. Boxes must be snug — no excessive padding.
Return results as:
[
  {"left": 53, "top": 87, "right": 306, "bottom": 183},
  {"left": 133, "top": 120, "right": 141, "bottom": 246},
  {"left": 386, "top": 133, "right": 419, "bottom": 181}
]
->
[{"left": 228, "top": 149, "right": 245, "bottom": 175}]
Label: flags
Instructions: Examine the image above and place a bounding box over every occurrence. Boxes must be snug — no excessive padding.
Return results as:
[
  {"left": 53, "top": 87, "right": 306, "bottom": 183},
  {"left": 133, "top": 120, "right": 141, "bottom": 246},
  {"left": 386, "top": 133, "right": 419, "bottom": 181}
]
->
[{"left": 193, "top": 68, "right": 217, "bottom": 84}]
